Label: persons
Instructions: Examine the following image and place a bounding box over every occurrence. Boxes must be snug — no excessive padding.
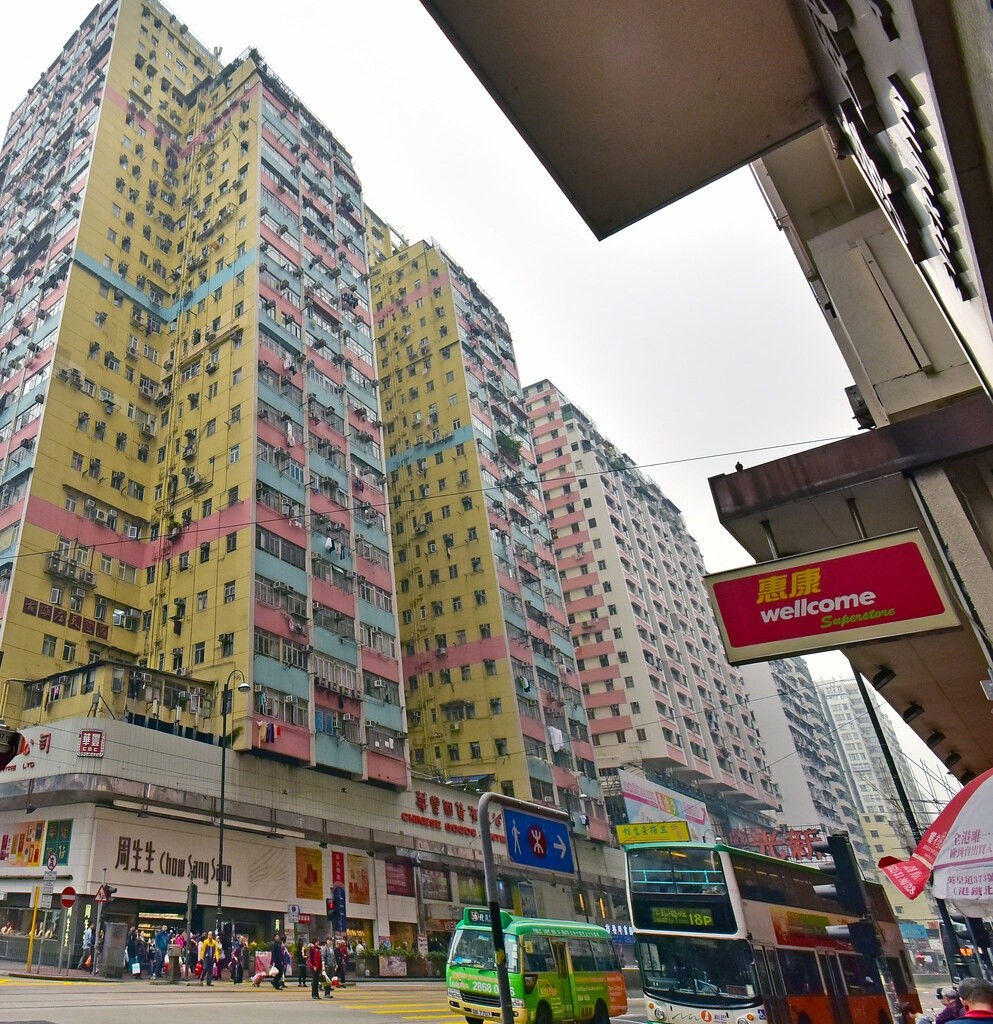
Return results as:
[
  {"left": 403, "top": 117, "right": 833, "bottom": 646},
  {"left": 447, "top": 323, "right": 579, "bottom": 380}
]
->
[
  {"left": 0, "top": 917, "right": 374, "bottom": 1001},
  {"left": 899, "top": 976, "right": 993, "bottom": 1022}
]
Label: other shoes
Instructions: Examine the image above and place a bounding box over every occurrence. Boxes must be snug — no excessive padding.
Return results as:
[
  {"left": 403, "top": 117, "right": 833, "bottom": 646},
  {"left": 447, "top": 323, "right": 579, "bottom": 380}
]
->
[
  {"left": 338, "top": 984, "right": 346, "bottom": 988},
  {"left": 311, "top": 995, "right": 322, "bottom": 1000},
  {"left": 77, "top": 965, "right": 83, "bottom": 970},
  {"left": 282, "top": 985, "right": 288, "bottom": 988},
  {"left": 324, "top": 995, "right": 333, "bottom": 998},
  {"left": 303, "top": 984, "right": 307, "bottom": 987},
  {"left": 272, "top": 983, "right": 283, "bottom": 990},
  {"left": 133, "top": 975, "right": 163, "bottom": 980},
  {"left": 298, "top": 984, "right": 302, "bottom": 987},
  {"left": 200, "top": 977, "right": 248, "bottom": 986},
  {"left": 319, "top": 989, "right": 324, "bottom": 991},
  {"left": 329, "top": 988, "right": 335, "bottom": 991}
]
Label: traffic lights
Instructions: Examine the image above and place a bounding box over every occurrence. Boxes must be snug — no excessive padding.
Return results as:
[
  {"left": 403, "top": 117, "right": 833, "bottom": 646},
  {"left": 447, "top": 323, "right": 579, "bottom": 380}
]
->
[
  {"left": 950, "top": 912, "right": 975, "bottom": 947},
  {"left": 326, "top": 899, "right": 334, "bottom": 922},
  {"left": 102, "top": 884, "right": 118, "bottom": 907}
]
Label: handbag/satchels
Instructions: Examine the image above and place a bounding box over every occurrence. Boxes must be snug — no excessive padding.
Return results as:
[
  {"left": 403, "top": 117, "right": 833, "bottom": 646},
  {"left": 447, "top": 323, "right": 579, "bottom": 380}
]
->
[
  {"left": 85, "top": 954, "right": 92, "bottom": 968},
  {"left": 211, "top": 963, "right": 218, "bottom": 977},
  {"left": 192, "top": 961, "right": 202, "bottom": 976},
  {"left": 318, "top": 971, "right": 332, "bottom": 986},
  {"left": 132, "top": 963, "right": 140, "bottom": 974},
  {"left": 268, "top": 964, "right": 279, "bottom": 977},
  {"left": 279, "top": 972, "right": 285, "bottom": 983},
  {"left": 331, "top": 976, "right": 339, "bottom": 987},
  {"left": 124, "top": 946, "right": 129, "bottom": 962}
]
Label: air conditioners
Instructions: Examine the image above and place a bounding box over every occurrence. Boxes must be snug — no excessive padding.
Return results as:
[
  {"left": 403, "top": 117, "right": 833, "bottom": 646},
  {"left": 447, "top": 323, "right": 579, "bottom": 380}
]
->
[{"left": 0, "top": 11, "right": 821, "bottom": 823}]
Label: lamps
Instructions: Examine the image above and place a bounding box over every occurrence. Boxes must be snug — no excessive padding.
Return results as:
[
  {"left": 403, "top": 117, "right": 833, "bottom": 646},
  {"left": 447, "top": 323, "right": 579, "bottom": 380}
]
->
[
  {"left": 366, "top": 850, "right": 375, "bottom": 857},
  {"left": 25, "top": 802, "right": 38, "bottom": 817},
  {"left": 413, "top": 858, "right": 422, "bottom": 866},
  {"left": 945, "top": 750, "right": 962, "bottom": 769},
  {"left": 598, "top": 886, "right": 608, "bottom": 895},
  {"left": 496, "top": 873, "right": 503, "bottom": 880},
  {"left": 550, "top": 882, "right": 557, "bottom": 888},
  {"left": 960, "top": 768, "right": 977, "bottom": 786},
  {"left": 927, "top": 728, "right": 946, "bottom": 749},
  {"left": 871, "top": 663, "right": 896, "bottom": 692},
  {"left": 136, "top": 809, "right": 150, "bottom": 819},
  {"left": 441, "top": 862, "right": 451, "bottom": 873},
  {"left": 318, "top": 842, "right": 328, "bottom": 848},
  {"left": 266, "top": 832, "right": 277, "bottom": 840},
  {"left": 903, "top": 700, "right": 926, "bottom": 723}
]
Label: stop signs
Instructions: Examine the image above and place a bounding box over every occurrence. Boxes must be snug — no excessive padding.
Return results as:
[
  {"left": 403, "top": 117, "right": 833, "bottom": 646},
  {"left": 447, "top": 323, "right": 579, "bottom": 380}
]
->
[{"left": 61, "top": 887, "right": 77, "bottom": 908}]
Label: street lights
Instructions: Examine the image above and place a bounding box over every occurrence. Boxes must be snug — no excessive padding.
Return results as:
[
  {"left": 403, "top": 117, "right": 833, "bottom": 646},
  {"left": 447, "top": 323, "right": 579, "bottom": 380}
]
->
[
  {"left": 215, "top": 668, "right": 251, "bottom": 940},
  {"left": 562, "top": 783, "right": 591, "bottom": 926}
]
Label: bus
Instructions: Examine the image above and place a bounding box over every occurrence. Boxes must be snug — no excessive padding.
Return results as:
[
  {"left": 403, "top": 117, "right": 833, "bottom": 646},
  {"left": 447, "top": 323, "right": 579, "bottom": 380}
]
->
[
  {"left": 445, "top": 907, "right": 627, "bottom": 1024},
  {"left": 624, "top": 840, "right": 924, "bottom": 1024}
]
